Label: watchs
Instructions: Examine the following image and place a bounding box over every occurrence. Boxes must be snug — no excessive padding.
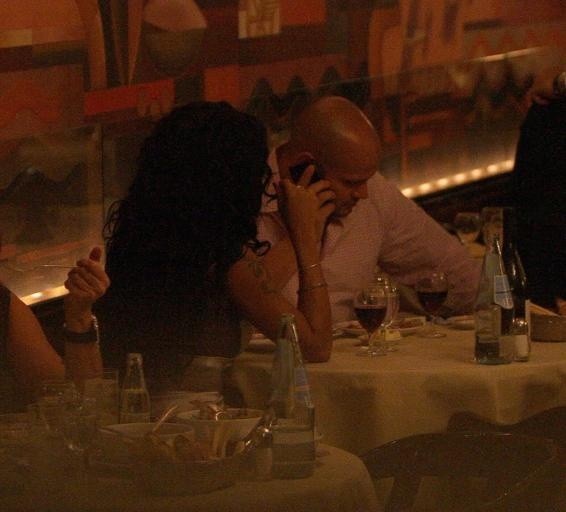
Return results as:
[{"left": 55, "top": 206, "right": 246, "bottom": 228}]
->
[{"left": 61, "top": 314, "right": 100, "bottom": 344}]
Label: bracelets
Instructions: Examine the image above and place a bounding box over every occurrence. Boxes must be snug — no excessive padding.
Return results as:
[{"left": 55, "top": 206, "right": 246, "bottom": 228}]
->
[
  {"left": 551, "top": 73, "right": 565, "bottom": 96},
  {"left": 295, "top": 259, "right": 322, "bottom": 273},
  {"left": 295, "top": 281, "right": 328, "bottom": 294}
]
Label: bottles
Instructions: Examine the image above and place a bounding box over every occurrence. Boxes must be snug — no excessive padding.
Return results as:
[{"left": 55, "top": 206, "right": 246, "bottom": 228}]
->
[
  {"left": 269, "top": 313, "right": 315, "bottom": 478},
  {"left": 119, "top": 352, "right": 151, "bottom": 422},
  {"left": 471, "top": 231, "right": 531, "bottom": 363}
]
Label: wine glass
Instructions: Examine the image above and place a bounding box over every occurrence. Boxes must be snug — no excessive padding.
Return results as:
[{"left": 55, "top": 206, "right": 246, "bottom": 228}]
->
[{"left": 352, "top": 270, "right": 450, "bottom": 356}]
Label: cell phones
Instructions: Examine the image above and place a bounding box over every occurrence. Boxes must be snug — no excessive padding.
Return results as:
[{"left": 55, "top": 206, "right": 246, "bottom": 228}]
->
[{"left": 288, "top": 158, "right": 332, "bottom": 208}]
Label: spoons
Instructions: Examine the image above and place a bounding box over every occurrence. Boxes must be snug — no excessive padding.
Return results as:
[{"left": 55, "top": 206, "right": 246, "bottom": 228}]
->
[{"left": 6, "top": 264, "right": 80, "bottom": 272}]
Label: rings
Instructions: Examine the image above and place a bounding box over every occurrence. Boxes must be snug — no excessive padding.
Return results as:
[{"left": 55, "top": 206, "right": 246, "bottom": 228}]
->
[{"left": 304, "top": 187, "right": 317, "bottom": 197}]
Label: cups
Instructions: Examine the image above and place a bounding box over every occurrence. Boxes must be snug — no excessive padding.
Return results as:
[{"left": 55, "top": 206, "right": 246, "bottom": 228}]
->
[{"left": 28, "top": 377, "right": 119, "bottom": 455}]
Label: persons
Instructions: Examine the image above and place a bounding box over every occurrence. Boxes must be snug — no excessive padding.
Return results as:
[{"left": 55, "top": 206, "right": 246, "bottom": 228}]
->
[
  {"left": 243, "top": 95, "right": 487, "bottom": 326},
  {"left": 0, "top": 245, "right": 111, "bottom": 406},
  {"left": 530, "top": 69, "right": 565, "bottom": 106},
  {"left": 90, "top": 98, "right": 337, "bottom": 385}
]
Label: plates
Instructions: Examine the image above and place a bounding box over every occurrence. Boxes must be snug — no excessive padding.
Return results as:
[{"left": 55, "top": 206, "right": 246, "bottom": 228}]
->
[
  {"left": 339, "top": 315, "right": 427, "bottom": 335},
  {"left": 448, "top": 316, "right": 474, "bottom": 330}
]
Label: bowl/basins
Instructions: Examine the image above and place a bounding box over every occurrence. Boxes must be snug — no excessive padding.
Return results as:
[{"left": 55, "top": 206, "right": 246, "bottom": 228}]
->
[{"left": 100, "top": 391, "right": 265, "bottom": 463}]
